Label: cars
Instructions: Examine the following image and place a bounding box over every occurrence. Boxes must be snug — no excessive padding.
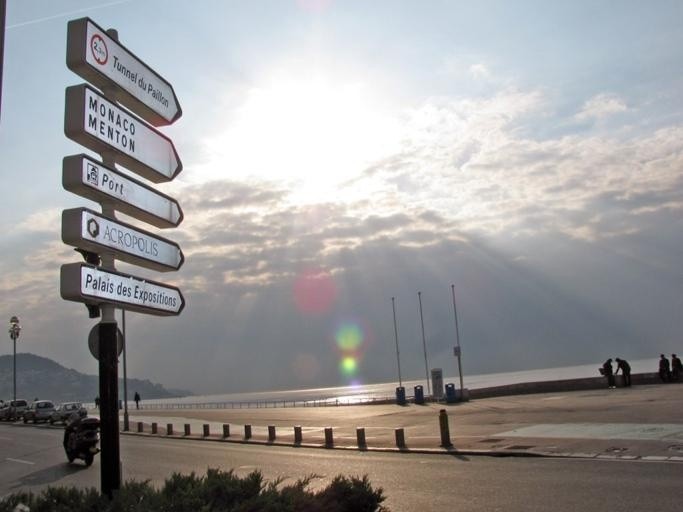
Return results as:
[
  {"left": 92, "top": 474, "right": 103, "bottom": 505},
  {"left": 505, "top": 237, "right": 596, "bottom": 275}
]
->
[{"left": 0, "top": 399, "right": 82, "bottom": 424}]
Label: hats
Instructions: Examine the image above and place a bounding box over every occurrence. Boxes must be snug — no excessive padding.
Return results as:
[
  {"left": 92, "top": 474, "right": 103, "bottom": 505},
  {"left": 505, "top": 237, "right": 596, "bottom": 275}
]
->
[{"left": 76, "top": 407, "right": 87, "bottom": 416}]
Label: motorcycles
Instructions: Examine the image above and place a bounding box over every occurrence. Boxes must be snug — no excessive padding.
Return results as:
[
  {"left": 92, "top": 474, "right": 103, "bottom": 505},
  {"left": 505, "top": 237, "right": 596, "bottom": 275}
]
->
[{"left": 63, "top": 418, "right": 100, "bottom": 467}]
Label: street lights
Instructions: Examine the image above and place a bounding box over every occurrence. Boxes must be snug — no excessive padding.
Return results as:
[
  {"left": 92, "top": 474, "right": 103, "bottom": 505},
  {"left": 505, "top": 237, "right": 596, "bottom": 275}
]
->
[{"left": 9, "top": 316, "right": 22, "bottom": 398}]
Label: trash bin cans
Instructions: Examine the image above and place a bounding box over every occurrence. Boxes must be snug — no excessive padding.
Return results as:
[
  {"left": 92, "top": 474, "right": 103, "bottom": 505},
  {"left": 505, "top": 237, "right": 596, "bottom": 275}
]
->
[
  {"left": 414, "top": 385, "right": 424, "bottom": 404},
  {"left": 119, "top": 400, "right": 121, "bottom": 409},
  {"left": 444, "top": 383, "right": 456, "bottom": 402},
  {"left": 396, "top": 387, "right": 405, "bottom": 404}
]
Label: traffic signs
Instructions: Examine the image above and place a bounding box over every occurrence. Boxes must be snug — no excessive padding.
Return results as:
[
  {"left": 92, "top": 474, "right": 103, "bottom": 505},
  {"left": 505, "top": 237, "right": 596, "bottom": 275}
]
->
[{"left": 60, "top": 16, "right": 184, "bottom": 316}]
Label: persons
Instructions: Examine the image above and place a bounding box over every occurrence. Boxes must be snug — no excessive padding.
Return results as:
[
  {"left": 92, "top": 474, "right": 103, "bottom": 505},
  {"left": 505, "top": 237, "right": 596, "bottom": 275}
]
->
[
  {"left": 613, "top": 357, "right": 631, "bottom": 387},
  {"left": 671, "top": 354, "right": 683, "bottom": 383},
  {"left": 602, "top": 358, "right": 616, "bottom": 389},
  {"left": 133, "top": 391, "right": 140, "bottom": 409},
  {"left": 658, "top": 353, "right": 672, "bottom": 384},
  {"left": 93, "top": 394, "right": 99, "bottom": 408}
]
